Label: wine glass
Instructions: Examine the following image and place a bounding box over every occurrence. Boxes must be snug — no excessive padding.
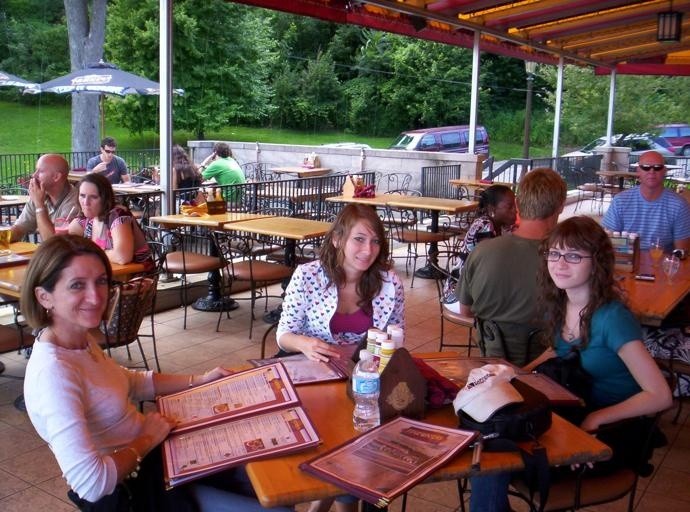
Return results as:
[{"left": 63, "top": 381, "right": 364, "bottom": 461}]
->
[
  {"left": 661, "top": 257, "right": 680, "bottom": 284},
  {"left": 650, "top": 243, "right": 664, "bottom": 269}
]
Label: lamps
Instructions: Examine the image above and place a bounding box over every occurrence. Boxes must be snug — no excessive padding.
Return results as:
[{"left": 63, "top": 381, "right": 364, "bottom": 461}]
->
[
  {"left": 410, "top": 16, "right": 428, "bottom": 33},
  {"left": 656, "top": 0, "right": 683, "bottom": 44}
]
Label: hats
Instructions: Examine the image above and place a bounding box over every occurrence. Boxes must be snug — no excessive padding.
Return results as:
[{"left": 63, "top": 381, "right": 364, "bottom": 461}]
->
[{"left": 453, "top": 364, "right": 524, "bottom": 423}]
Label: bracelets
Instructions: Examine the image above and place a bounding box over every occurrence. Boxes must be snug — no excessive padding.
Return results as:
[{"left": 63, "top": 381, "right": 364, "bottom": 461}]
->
[
  {"left": 189, "top": 375, "right": 194, "bottom": 387},
  {"left": 114, "top": 446, "right": 142, "bottom": 479},
  {"left": 35, "top": 206, "right": 47, "bottom": 214}
]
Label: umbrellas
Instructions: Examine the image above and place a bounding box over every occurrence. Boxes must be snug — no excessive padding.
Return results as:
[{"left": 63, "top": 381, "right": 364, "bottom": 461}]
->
[
  {"left": 21, "top": 56, "right": 186, "bottom": 138},
  {"left": 0, "top": 71, "right": 38, "bottom": 88}
]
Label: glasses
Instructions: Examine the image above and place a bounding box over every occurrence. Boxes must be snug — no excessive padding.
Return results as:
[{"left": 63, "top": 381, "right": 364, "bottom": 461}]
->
[
  {"left": 639, "top": 164, "right": 664, "bottom": 170},
  {"left": 105, "top": 150, "right": 115, "bottom": 153},
  {"left": 544, "top": 251, "right": 591, "bottom": 264}
]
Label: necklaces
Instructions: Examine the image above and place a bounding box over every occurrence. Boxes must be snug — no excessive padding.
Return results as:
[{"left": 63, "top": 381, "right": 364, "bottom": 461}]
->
[
  {"left": 566, "top": 318, "right": 578, "bottom": 340},
  {"left": 83, "top": 340, "right": 101, "bottom": 363}
]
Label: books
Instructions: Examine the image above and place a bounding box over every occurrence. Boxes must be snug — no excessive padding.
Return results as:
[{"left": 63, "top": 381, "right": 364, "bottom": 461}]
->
[
  {"left": 153, "top": 360, "right": 326, "bottom": 492},
  {"left": 423, "top": 356, "right": 586, "bottom": 407},
  {"left": 297, "top": 415, "right": 481, "bottom": 510},
  {"left": 248, "top": 352, "right": 356, "bottom": 385}
]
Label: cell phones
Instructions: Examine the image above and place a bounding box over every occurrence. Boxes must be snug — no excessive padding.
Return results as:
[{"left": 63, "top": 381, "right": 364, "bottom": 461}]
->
[
  {"left": 212, "top": 149, "right": 216, "bottom": 160},
  {"left": 636, "top": 273, "right": 655, "bottom": 280}
]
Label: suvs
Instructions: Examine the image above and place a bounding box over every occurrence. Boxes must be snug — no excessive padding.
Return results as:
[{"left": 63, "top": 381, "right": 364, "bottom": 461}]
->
[
  {"left": 384, "top": 119, "right": 493, "bottom": 167},
  {"left": 644, "top": 120, "right": 690, "bottom": 158}
]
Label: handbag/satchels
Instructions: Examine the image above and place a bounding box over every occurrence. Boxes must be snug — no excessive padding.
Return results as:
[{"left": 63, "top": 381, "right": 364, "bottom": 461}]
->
[{"left": 458, "top": 378, "right": 551, "bottom": 451}]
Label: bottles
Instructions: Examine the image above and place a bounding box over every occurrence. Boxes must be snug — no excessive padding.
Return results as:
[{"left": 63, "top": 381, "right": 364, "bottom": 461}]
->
[
  {"left": 196, "top": 187, "right": 223, "bottom": 203},
  {"left": 0, "top": 227, "right": 11, "bottom": 256},
  {"left": 55, "top": 218, "right": 68, "bottom": 235},
  {"left": 302, "top": 152, "right": 321, "bottom": 169},
  {"left": 352, "top": 348, "right": 381, "bottom": 432},
  {"left": 349, "top": 173, "right": 363, "bottom": 191},
  {"left": 368, "top": 323, "right": 405, "bottom": 373},
  {"left": 602, "top": 227, "right": 638, "bottom": 268},
  {"left": 140, "top": 166, "right": 160, "bottom": 184}
]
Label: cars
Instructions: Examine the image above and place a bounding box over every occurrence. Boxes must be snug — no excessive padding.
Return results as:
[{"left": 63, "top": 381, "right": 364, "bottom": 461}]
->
[{"left": 556, "top": 132, "right": 680, "bottom": 175}]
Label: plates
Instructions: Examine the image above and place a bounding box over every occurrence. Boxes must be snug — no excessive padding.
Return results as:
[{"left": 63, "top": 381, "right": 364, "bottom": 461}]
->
[{"left": 10, "top": 241, "right": 39, "bottom": 254}]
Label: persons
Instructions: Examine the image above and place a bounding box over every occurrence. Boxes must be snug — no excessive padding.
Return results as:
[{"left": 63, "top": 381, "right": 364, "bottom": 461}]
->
[
  {"left": 6, "top": 154, "right": 83, "bottom": 244},
  {"left": 599, "top": 151, "right": 689, "bottom": 260},
  {"left": 151, "top": 144, "right": 202, "bottom": 217},
  {"left": 198, "top": 140, "right": 247, "bottom": 209},
  {"left": 454, "top": 166, "right": 568, "bottom": 367},
  {"left": 437, "top": 185, "right": 518, "bottom": 315},
  {"left": 66, "top": 173, "right": 156, "bottom": 290},
  {"left": 85, "top": 137, "right": 130, "bottom": 183},
  {"left": 19, "top": 232, "right": 293, "bottom": 512},
  {"left": 275, "top": 202, "right": 407, "bottom": 363},
  {"left": 466, "top": 214, "right": 675, "bottom": 512}
]
